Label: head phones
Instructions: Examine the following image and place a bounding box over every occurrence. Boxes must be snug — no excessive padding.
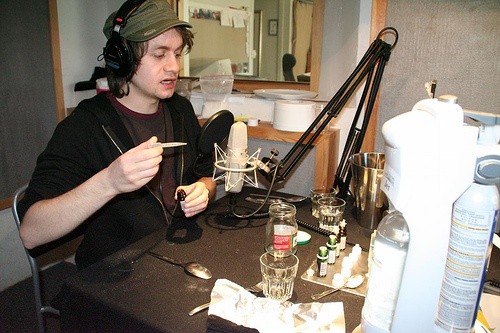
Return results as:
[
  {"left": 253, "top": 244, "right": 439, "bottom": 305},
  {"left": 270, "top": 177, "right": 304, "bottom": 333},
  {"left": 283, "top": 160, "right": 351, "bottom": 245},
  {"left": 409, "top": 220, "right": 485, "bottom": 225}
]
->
[{"left": 103, "top": 0, "right": 147, "bottom": 77}]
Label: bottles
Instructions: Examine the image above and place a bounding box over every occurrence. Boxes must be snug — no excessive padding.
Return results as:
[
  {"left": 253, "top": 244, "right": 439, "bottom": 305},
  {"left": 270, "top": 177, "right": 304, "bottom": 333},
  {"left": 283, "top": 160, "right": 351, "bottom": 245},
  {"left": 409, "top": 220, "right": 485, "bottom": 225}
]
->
[{"left": 264, "top": 202, "right": 299, "bottom": 256}]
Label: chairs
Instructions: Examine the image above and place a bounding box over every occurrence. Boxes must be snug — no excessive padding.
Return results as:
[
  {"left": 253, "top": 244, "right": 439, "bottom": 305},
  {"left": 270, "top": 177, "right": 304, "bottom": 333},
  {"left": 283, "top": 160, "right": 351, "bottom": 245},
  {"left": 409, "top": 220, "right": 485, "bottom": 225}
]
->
[{"left": 10, "top": 185, "right": 81, "bottom": 333}]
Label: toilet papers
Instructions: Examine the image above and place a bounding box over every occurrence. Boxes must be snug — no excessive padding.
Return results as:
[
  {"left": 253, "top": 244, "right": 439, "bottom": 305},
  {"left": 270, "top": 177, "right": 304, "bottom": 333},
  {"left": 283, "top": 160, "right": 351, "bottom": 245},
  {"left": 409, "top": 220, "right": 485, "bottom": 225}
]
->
[{"left": 270, "top": 98, "right": 316, "bottom": 133}]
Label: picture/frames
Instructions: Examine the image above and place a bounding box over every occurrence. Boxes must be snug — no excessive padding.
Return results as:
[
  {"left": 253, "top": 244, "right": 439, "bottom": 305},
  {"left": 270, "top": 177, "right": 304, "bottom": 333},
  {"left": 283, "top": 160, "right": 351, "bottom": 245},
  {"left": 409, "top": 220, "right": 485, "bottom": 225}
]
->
[
  {"left": 171, "top": 0, "right": 261, "bottom": 77},
  {"left": 269, "top": 19, "right": 278, "bottom": 36}
]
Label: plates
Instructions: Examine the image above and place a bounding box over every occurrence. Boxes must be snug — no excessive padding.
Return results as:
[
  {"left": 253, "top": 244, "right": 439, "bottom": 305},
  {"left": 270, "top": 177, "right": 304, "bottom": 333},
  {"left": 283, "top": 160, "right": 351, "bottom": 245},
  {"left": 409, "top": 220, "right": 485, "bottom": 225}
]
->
[{"left": 253, "top": 89, "right": 318, "bottom": 100}]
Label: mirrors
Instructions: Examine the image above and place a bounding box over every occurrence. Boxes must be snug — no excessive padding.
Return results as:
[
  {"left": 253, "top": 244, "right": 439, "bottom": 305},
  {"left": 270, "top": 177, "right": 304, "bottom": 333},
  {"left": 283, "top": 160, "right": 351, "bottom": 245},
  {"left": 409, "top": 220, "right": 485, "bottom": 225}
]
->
[{"left": 170, "top": 1, "right": 325, "bottom": 93}]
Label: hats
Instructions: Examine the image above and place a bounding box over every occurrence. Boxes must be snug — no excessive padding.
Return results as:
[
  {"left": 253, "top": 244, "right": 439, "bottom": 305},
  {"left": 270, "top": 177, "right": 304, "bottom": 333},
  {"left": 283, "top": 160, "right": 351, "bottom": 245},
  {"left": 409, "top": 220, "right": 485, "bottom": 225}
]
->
[{"left": 103, "top": 0, "right": 192, "bottom": 42}]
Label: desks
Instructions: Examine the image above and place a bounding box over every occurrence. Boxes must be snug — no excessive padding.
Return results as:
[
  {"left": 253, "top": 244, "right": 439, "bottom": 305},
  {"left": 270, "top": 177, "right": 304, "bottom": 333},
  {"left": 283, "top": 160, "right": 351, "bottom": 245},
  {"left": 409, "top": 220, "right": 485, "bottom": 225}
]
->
[
  {"left": 195, "top": 116, "right": 339, "bottom": 196},
  {"left": 61, "top": 183, "right": 371, "bottom": 333}
]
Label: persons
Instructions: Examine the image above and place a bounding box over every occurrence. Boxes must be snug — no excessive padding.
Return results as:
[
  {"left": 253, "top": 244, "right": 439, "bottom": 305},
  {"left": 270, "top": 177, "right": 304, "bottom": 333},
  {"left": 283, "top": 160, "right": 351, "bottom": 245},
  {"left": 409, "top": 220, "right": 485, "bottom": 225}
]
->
[{"left": 17, "top": 0, "right": 218, "bottom": 274}]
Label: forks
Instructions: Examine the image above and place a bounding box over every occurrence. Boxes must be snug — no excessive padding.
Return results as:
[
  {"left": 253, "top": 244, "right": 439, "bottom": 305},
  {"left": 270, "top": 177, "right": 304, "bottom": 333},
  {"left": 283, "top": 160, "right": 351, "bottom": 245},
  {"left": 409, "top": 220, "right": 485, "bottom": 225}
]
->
[{"left": 187, "top": 281, "right": 263, "bottom": 317}]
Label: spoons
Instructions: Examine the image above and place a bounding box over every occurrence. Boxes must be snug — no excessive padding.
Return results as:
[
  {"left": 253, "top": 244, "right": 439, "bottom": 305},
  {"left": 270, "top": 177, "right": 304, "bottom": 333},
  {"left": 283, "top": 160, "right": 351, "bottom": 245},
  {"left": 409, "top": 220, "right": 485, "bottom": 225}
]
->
[
  {"left": 312, "top": 272, "right": 365, "bottom": 300},
  {"left": 145, "top": 249, "right": 212, "bottom": 280}
]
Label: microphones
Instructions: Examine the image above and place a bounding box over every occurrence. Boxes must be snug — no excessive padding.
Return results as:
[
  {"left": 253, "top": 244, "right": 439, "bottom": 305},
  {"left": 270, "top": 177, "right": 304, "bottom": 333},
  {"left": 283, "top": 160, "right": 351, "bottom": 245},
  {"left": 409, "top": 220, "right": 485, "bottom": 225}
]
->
[{"left": 225, "top": 121, "right": 248, "bottom": 193}]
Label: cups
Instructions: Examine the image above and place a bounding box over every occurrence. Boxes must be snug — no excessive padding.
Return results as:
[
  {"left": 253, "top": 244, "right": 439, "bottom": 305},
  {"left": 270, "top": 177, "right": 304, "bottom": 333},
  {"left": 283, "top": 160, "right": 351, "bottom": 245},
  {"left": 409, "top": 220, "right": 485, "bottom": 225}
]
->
[
  {"left": 349, "top": 152, "right": 389, "bottom": 230},
  {"left": 310, "top": 185, "right": 336, "bottom": 217},
  {"left": 260, "top": 251, "right": 299, "bottom": 301},
  {"left": 317, "top": 198, "right": 347, "bottom": 232}
]
